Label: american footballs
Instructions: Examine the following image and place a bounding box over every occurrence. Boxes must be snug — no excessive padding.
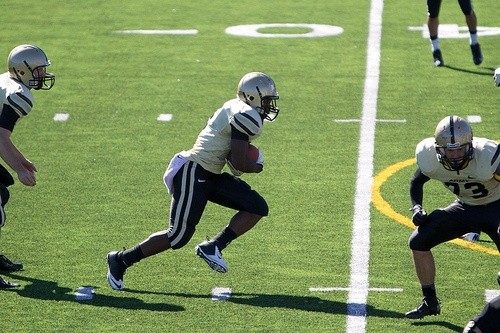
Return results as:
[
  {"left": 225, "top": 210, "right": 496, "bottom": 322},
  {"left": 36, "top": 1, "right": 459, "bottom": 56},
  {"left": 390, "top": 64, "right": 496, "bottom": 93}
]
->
[{"left": 227, "top": 143, "right": 259, "bottom": 169}]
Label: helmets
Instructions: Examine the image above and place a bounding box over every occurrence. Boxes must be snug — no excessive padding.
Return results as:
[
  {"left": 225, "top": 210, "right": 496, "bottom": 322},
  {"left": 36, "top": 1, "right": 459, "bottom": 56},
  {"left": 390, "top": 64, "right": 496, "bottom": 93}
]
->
[
  {"left": 238, "top": 72, "right": 279, "bottom": 118},
  {"left": 434, "top": 116, "right": 473, "bottom": 148},
  {"left": 8, "top": 45, "right": 51, "bottom": 89}
]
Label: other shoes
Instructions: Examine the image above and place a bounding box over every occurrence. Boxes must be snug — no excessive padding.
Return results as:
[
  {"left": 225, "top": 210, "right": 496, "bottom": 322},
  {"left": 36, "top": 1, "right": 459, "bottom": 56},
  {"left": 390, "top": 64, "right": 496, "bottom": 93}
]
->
[
  {"left": 0, "top": 254, "right": 22, "bottom": 275},
  {"left": 0, "top": 276, "right": 21, "bottom": 290}
]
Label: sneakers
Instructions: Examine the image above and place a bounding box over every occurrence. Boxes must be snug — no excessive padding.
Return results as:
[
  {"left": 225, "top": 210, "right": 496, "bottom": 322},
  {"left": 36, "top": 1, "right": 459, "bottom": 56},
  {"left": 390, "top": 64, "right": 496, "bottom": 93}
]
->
[
  {"left": 433, "top": 48, "right": 443, "bottom": 67},
  {"left": 470, "top": 43, "right": 482, "bottom": 65},
  {"left": 404, "top": 295, "right": 440, "bottom": 318},
  {"left": 106, "top": 247, "right": 127, "bottom": 290},
  {"left": 195, "top": 234, "right": 229, "bottom": 273},
  {"left": 461, "top": 231, "right": 480, "bottom": 242}
]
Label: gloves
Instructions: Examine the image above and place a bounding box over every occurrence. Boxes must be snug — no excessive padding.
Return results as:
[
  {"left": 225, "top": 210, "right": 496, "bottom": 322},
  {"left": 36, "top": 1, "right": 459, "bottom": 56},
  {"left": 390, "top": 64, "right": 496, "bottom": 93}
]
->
[
  {"left": 225, "top": 158, "right": 244, "bottom": 176},
  {"left": 409, "top": 205, "right": 428, "bottom": 226},
  {"left": 493, "top": 67, "right": 500, "bottom": 87},
  {"left": 256, "top": 147, "right": 264, "bottom": 168}
]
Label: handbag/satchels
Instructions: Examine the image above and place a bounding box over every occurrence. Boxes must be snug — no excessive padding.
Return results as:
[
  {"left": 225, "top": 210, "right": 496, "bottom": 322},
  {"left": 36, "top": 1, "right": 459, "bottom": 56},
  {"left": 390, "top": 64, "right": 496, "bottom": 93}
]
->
[{"left": 164, "top": 150, "right": 192, "bottom": 196}]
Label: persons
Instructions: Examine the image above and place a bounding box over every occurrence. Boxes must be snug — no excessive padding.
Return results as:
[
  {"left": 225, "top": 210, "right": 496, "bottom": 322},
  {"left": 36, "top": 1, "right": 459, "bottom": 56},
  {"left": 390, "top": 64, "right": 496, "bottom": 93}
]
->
[
  {"left": 426, "top": 0, "right": 486, "bottom": 67},
  {"left": 405, "top": 116, "right": 500, "bottom": 320},
  {"left": 106, "top": 71, "right": 281, "bottom": 293},
  {"left": 0, "top": 44, "right": 56, "bottom": 289},
  {"left": 461, "top": 276, "right": 500, "bottom": 333},
  {"left": 493, "top": 68, "right": 500, "bottom": 87}
]
